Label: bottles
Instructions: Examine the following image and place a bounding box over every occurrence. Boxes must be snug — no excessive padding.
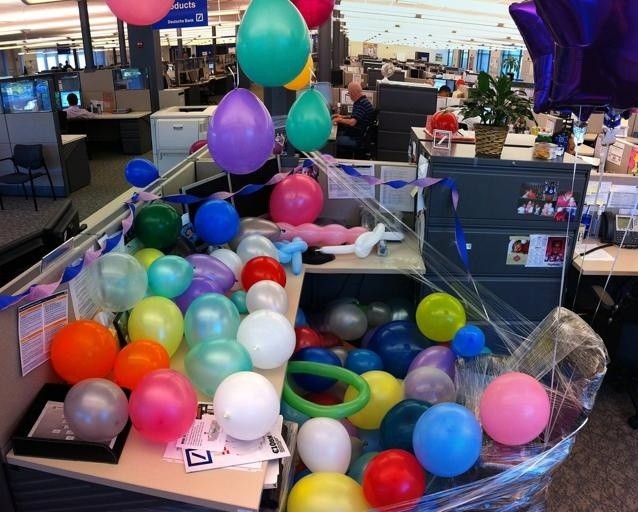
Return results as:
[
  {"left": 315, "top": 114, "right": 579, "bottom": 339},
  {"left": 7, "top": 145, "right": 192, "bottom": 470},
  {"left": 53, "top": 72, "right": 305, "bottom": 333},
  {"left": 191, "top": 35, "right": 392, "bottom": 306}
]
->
[{"left": 581, "top": 210, "right": 602, "bottom": 239}]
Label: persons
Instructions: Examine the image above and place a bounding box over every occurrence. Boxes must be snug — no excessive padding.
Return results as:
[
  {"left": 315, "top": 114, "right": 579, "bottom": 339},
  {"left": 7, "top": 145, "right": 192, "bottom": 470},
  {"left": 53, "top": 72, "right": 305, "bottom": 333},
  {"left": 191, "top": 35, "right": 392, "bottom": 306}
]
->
[
  {"left": 511, "top": 187, "right": 576, "bottom": 261},
  {"left": 58, "top": 61, "right": 73, "bottom": 71},
  {"left": 162, "top": 63, "right": 172, "bottom": 87},
  {"left": 63, "top": 93, "right": 94, "bottom": 120},
  {"left": 327, "top": 81, "right": 375, "bottom": 158},
  {"left": 439, "top": 85, "right": 452, "bottom": 96}
]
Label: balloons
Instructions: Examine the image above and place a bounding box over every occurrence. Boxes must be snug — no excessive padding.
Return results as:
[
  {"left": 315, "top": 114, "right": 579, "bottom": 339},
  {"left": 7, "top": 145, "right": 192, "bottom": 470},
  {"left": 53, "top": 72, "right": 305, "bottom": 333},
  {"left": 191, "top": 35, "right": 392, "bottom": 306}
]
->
[
  {"left": 49, "top": 155, "right": 551, "bottom": 511},
  {"left": 106, "top": 1, "right": 173, "bottom": 26},
  {"left": 189, "top": 139, "right": 207, "bottom": 155},
  {"left": 508, "top": 0, "right": 638, "bottom": 128},
  {"left": 208, "top": 0, "right": 333, "bottom": 174}
]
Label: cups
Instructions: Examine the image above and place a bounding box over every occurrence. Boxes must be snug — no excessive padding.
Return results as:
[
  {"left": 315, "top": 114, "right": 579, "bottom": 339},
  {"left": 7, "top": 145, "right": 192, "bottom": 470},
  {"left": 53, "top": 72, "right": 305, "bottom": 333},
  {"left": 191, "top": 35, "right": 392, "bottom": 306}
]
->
[
  {"left": 532, "top": 133, "right": 553, "bottom": 159},
  {"left": 97, "top": 104, "right": 101, "bottom": 114},
  {"left": 575, "top": 224, "right": 586, "bottom": 244}
]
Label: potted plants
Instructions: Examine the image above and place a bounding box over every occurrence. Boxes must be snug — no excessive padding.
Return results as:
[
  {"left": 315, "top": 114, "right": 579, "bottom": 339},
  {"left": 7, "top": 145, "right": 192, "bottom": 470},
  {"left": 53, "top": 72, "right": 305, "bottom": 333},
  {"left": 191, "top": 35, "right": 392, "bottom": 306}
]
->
[{"left": 457, "top": 69, "right": 536, "bottom": 157}]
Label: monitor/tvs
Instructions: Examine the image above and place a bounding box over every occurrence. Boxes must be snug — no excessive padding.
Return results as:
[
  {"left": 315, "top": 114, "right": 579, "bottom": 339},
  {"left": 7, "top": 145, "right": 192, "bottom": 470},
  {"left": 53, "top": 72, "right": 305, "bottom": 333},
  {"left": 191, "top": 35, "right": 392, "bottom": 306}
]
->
[
  {"left": 59, "top": 89, "right": 83, "bottom": 109},
  {"left": 208, "top": 63, "right": 215, "bottom": 69},
  {"left": 295, "top": 81, "right": 331, "bottom": 105},
  {"left": 447, "top": 80, "right": 455, "bottom": 94},
  {"left": 433, "top": 78, "right": 447, "bottom": 92}
]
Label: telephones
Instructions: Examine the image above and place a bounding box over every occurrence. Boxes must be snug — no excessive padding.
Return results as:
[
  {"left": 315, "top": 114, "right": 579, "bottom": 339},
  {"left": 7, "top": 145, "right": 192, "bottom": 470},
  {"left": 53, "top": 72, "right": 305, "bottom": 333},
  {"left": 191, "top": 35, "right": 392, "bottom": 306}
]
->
[{"left": 600, "top": 211, "right": 638, "bottom": 245}]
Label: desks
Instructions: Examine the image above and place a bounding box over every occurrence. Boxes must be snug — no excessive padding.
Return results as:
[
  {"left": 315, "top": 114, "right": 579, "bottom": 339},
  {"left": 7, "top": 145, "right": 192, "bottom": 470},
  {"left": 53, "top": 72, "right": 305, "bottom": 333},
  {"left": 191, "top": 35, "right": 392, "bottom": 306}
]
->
[{"left": 1, "top": 108, "right": 638, "bottom": 512}]
